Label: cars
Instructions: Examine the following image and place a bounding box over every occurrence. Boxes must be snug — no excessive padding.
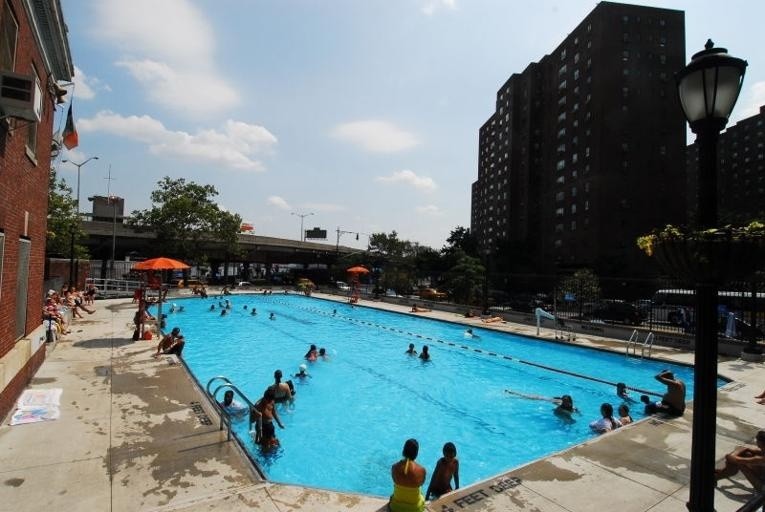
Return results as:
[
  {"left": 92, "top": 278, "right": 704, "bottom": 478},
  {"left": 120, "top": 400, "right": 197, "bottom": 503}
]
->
[
  {"left": 231, "top": 270, "right": 350, "bottom": 293},
  {"left": 373, "top": 281, "right": 654, "bottom": 327},
  {"left": 686, "top": 314, "right": 763, "bottom": 341}
]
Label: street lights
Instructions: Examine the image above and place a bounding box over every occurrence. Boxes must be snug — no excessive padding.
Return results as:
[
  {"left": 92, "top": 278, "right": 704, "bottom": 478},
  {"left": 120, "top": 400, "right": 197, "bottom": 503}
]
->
[
  {"left": 291, "top": 212, "right": 315, "bottom": 242},
  {"left": 88, "top": 192, "right": 118, "bottom": 269},
  {"left": 63, "top": 157, "right": 99, "bottom": 291},
  {"left": 670, "top": 37, "right": 749, "bottom": 512},
  {"left": 334, "top": 227, "right": 360, "bottom": 261},
  {"left": 479, "top": 248, "right": 495, "bottom": 315}
]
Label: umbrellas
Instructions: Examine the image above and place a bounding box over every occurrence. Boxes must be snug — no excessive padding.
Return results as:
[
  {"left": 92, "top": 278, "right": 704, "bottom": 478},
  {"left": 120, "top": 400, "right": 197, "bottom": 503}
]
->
[{"left": 133, "top": 256, "right": 190, "bottom": 299}]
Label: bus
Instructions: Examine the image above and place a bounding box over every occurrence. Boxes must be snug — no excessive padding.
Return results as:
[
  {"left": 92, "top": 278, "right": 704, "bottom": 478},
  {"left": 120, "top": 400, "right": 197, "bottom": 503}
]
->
[{"left": 652, "top": 288, "right": 765, "bottom": 338}]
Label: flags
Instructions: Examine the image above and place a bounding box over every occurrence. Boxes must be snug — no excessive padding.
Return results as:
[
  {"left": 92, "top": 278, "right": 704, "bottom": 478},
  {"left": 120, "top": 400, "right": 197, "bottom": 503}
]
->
[{"left": 62, "top": 98, "right": 78, "bottom": 150}]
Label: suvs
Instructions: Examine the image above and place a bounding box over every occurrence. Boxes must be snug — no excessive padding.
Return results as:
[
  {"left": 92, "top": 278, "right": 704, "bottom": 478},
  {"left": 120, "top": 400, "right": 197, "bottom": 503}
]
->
[{"left": 178, "top": 280, "right": 208, "bottom": 289}]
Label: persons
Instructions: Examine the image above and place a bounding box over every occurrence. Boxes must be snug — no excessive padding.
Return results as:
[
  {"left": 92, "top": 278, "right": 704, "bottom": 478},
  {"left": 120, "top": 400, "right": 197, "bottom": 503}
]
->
[
  {"left": 504, "top": 368, "right": 688, "bottom": 435},
  {"left": 753, "top": 385, "right": 765, "bottom": 407},
  {"left": 249, "top": 363, "right": 313, "bottom": 456},
  {"left": 426, "top": 442, "right": 461, "bottom": 501},
  {"left": 40, "top": 266, "right": 506, "bottom": 362},
  {"left": 391, "top": 437, "right": 426, "bottom": 509},
  {"left": 219, "top": 391, "right": 245, "bottom": 418},
  {"left": 715, "top": 430, "right": 765, "bottom": 492}
]
